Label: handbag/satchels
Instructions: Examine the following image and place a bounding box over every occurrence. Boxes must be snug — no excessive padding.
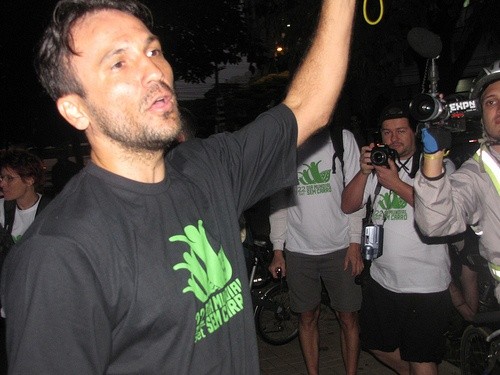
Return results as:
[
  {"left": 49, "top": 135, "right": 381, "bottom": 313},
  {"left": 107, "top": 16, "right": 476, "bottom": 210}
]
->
[{"left": 361, "top": 224, "right": 383, "bottom": 260}]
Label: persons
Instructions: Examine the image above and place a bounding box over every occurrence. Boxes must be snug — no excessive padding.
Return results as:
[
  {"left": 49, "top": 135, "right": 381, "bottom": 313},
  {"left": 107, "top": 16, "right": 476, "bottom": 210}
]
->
[
  {"left": 340, "top": 105, "right": 456, "bottom": 375},
  {"left": 414, "top": 70, "right": 500, "bottom": 320},
  {"left": 269, "top": 113, "right": 367, "bottom": 375},
  {"left": 0, "top": 0, "right": 356, "bottom": 375}
]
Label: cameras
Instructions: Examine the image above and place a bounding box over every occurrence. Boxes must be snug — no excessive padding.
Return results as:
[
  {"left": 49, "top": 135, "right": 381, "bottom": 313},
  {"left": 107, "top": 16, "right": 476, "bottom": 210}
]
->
[
  {"left": 407, "top": 27, "right": 482, "bottom": 133},
  {"left": 366, "top": 144, "right": 396, "bottom": 169},
  {"left": 360, "top": 224, "right": 383, "bottom": 262}
]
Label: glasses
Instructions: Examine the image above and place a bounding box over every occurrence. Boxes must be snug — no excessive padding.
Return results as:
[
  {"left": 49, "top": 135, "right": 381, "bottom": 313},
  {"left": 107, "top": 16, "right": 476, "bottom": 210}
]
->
[{"left": 0, "top": 172, "right": 28, "bottom": 182}]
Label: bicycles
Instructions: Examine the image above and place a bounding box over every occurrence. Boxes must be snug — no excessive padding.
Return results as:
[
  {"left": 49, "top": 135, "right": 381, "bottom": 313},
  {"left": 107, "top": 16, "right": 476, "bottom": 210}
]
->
[
  {"left": 458, "top": 315, "right": 499, "bottom": 375},
  {"left": 247, "top": 237, "right": 300, "bottom": 346}
]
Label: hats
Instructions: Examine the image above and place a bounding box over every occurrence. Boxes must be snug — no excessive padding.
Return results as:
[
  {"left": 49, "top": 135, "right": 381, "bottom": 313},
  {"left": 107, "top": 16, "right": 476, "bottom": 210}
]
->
[{"left": 469, "top": 61, "right": 500, "bottom": 100}]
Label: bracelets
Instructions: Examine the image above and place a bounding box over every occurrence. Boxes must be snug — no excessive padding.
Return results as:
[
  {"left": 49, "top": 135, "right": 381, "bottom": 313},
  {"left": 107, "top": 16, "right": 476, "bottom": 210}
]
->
[{"left": 422, "top": 150, "right": 449, "bottom": 160}]
